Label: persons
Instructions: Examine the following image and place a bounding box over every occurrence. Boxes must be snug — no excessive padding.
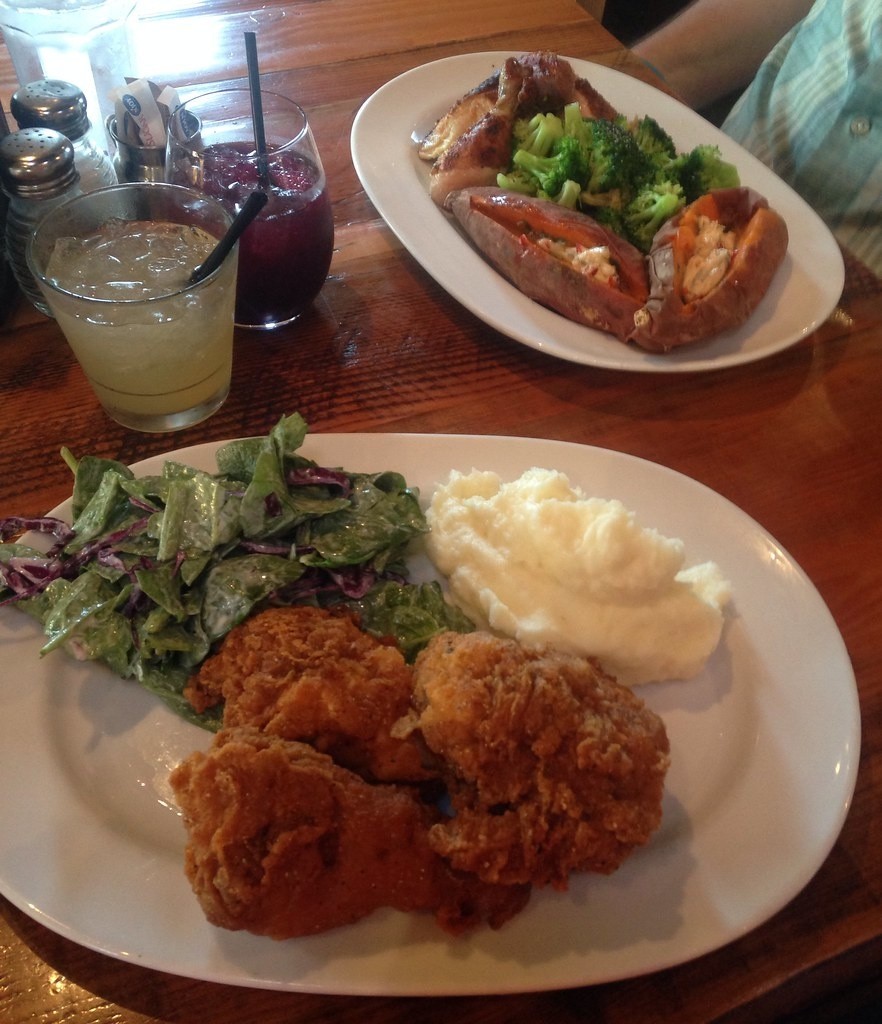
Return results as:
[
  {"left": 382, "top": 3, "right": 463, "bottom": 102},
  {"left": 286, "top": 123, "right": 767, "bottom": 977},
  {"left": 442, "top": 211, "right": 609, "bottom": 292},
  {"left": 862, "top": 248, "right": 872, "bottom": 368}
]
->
[{"left": 626, "top": 1, "right": 881, "bottom": 280}]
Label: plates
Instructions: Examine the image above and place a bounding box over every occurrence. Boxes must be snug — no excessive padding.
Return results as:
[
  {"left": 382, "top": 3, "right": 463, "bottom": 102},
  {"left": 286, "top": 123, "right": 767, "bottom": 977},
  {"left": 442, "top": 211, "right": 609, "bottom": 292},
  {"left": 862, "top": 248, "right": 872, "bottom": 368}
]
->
[
  {"left": 0, "top": 433, "right": 861, "bottom": 996},
  {"left": 350, "top": 50, "right": 846, "bottom": 375}
]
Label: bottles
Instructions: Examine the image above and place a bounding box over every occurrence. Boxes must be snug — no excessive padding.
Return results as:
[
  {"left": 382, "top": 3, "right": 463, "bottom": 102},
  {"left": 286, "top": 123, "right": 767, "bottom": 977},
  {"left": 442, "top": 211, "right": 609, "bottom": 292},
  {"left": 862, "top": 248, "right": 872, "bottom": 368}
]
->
[
  {"left": 10, "top": 79, "right": 119, "bottom": 194},
  {"left": 0, "top": 128, "right": 84, "bottom": 319}
]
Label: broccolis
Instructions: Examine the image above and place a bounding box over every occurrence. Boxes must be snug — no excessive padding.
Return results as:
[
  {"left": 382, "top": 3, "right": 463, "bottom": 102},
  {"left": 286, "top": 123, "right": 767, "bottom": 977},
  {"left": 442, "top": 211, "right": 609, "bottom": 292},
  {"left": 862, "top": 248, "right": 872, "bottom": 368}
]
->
[{"left": 495, "top": 101, "right": 744, "bottom": 251}]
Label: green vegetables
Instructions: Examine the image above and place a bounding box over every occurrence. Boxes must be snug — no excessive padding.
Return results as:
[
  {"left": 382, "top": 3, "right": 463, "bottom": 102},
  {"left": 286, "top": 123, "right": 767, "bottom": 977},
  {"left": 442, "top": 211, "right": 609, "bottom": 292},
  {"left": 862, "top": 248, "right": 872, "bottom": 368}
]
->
[{"left": 0, "top": 411, "right": 477, "bottom": 737}]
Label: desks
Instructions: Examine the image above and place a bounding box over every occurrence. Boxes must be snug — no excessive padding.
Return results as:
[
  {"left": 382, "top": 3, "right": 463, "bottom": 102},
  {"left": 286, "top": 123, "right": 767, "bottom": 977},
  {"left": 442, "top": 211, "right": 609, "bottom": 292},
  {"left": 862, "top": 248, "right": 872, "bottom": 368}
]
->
[{"left": 0, "top": 1, "right": 881, "bottom": 1024}]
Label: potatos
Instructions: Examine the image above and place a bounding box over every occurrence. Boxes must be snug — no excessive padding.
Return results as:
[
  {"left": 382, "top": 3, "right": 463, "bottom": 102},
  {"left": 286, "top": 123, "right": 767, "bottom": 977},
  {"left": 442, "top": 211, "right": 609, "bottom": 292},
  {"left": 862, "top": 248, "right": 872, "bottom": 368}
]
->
[
  {"left": 630, "top": 186, "right": 790, "bottom": 346},
  {"left": 445, "top": 188, "right": 651, "bottom": 342}
]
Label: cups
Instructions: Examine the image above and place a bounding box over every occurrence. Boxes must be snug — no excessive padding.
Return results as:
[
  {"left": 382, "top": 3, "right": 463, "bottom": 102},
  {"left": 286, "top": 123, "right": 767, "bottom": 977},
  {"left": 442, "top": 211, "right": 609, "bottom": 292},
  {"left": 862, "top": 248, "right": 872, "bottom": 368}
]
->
[
  {"left": 106, "top": 110, "right": 205, "bottom": 189},
  {"left": 163, "top": 89, "right": 334, "bottom": 332},
  {"left": 25, "top": 182, "right": 239, "bottom": 434}
]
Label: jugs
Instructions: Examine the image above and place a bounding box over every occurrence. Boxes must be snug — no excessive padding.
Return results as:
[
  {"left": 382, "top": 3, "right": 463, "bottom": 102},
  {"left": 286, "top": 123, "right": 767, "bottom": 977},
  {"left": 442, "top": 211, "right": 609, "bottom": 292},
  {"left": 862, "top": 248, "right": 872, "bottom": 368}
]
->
[{"left": 0, "top": 1, "right": 138, "bottom": 185}]
